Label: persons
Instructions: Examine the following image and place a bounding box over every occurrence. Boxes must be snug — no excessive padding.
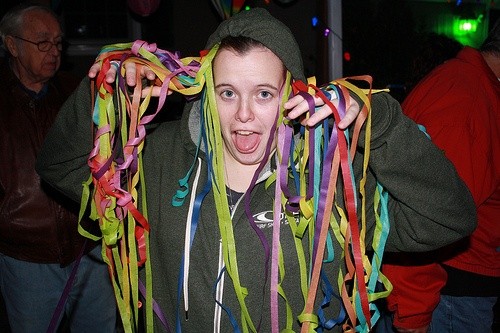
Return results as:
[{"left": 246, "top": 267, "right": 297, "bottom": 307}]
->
[
  {"left": 380, "top": 20, "right": 500, "bottom": 333},
  {"left": 35, "top": 7, "right": 477, "bottom": 333},
  {"left": 0, "top": 4, "right": 118, "bottom": 333}
]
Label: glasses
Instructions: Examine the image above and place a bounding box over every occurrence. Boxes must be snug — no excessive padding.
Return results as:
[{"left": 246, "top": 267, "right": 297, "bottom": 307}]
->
[{"left": 15, "top": 36, "right": 70, "bottom": 52}]
